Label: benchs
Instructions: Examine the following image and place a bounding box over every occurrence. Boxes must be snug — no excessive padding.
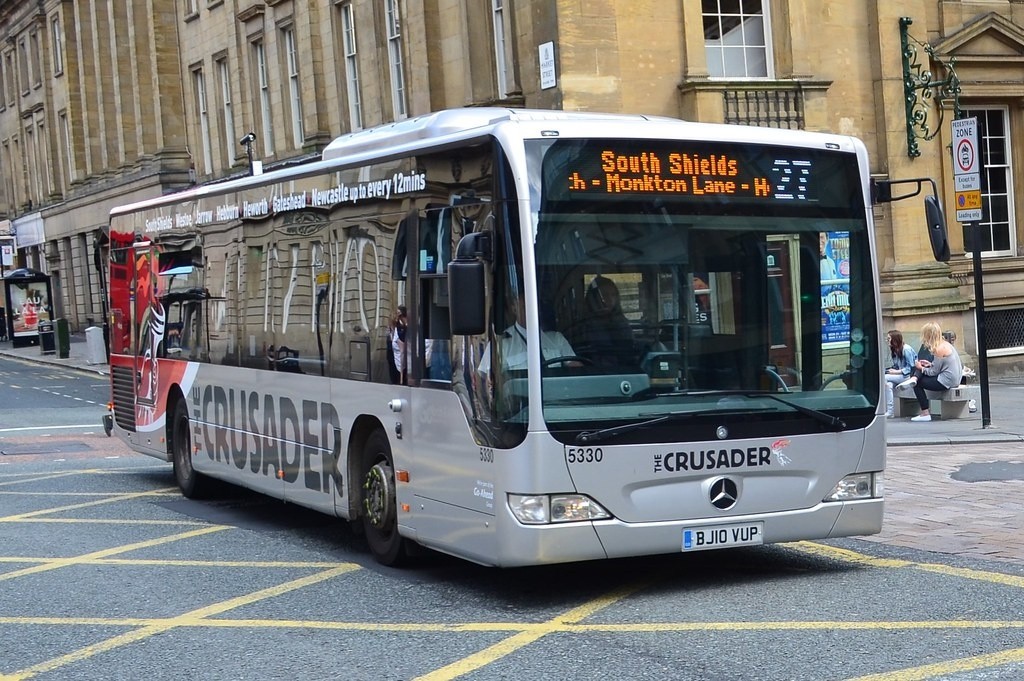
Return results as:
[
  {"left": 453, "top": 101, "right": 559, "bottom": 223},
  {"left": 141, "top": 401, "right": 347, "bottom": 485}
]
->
[{"left": 893, "top": 388, "right": 968, "bottom": 421}]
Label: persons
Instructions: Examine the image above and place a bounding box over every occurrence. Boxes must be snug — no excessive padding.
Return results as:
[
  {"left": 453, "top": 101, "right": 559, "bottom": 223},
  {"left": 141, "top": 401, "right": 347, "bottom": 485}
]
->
[
  {"left": 885, "top": 329, "right": 917, "bottom": 419},
  {"left": 895, "top": 322, "right": 963, "bottom": 422},
  {"left": 918, "top": 331, "right": 967, "bottom": 385},
  {"left": 574, "top": 275, "right": 635, "bottom": 360},
  {"left": 819, "top": 232, "right": 836, "bottom": 295},
  {"left": 477, "top": 285, "right": 581, "bottom": 407},
  {"left": 386, "top": 307, "right": 432, "bottom": 384}
]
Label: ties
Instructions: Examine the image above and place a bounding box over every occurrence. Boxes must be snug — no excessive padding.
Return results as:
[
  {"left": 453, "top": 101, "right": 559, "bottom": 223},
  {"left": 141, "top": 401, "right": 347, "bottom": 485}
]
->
[{"left": 541, "top": 353, "right": 547, "bottom": 368}]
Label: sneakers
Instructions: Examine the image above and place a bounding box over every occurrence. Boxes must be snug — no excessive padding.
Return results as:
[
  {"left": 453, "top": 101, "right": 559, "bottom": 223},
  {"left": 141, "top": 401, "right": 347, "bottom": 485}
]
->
[
  {"left": 895, "top": 379, "right": 917, "bottom": 392},
  {"left": 910, "top": 415, "right": 931, "bottom": 422}
]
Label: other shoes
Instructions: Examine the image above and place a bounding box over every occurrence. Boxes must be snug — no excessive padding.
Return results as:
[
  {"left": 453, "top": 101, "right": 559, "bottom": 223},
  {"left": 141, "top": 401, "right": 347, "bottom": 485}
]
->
[{"left": 885, "top": 411, "right": 894, "bottom": 419}]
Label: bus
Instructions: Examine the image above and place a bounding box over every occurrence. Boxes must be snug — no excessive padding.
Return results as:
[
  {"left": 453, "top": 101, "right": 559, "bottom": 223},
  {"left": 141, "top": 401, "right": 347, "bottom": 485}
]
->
[{"left": 108, "top": 107, "right": 952, "bottom": 569}]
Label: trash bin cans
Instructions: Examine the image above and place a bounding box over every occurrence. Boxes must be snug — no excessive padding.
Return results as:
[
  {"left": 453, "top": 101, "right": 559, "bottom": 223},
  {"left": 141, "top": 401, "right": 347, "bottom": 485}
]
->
[
  {"left": 37, "top": 322, "right": 56, "bottom": 355},
  {"left": 85, "top": 326, "right": 107, "bottom": 365}
]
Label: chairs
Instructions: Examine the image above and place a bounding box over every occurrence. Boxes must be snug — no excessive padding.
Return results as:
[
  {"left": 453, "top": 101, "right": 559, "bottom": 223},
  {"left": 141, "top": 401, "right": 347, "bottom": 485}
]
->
[{"left": 582, "top": 277, "right": 636, "bottom": 344}]
